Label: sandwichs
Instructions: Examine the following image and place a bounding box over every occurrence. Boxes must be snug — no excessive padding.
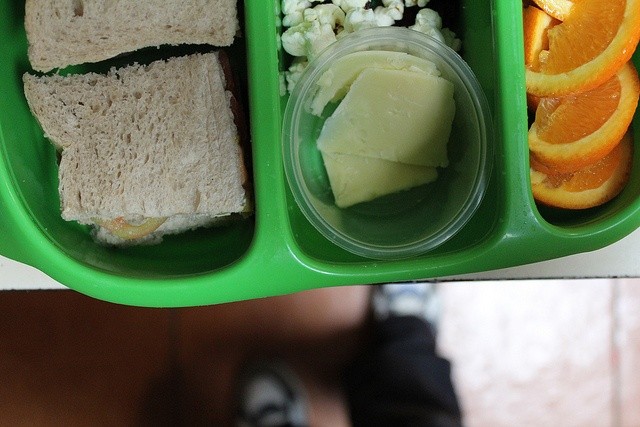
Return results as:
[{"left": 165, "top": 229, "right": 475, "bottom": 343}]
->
[
  {"left": 22, "top": 51, "right": 252, "bottom": 247},
  {"left": 24, "top": 0, "right": 240, "bottom": 74}
]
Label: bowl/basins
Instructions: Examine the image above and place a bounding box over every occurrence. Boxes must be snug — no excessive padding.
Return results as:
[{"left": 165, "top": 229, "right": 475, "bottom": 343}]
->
[{"left": 285, "top": 25, "right": 495, "bottom": 259}]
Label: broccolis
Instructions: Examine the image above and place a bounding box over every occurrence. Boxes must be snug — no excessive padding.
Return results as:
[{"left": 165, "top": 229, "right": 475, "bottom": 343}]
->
[{"left": 281, "top": 0, "right": 462, "bottom": 96}]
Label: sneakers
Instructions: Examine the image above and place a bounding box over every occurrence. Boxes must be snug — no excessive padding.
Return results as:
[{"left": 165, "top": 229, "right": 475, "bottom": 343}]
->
[
  {"left": 367, "top": 282, "right": 441, "bottom": 351},
  {"left": 230, "top": 355, "right": 309, "bottom": 426}
]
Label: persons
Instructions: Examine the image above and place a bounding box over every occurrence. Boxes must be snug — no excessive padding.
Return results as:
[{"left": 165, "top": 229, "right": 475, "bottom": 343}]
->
[{"left": 231, "top": 281, "right": 462, "bottom": 427}]
